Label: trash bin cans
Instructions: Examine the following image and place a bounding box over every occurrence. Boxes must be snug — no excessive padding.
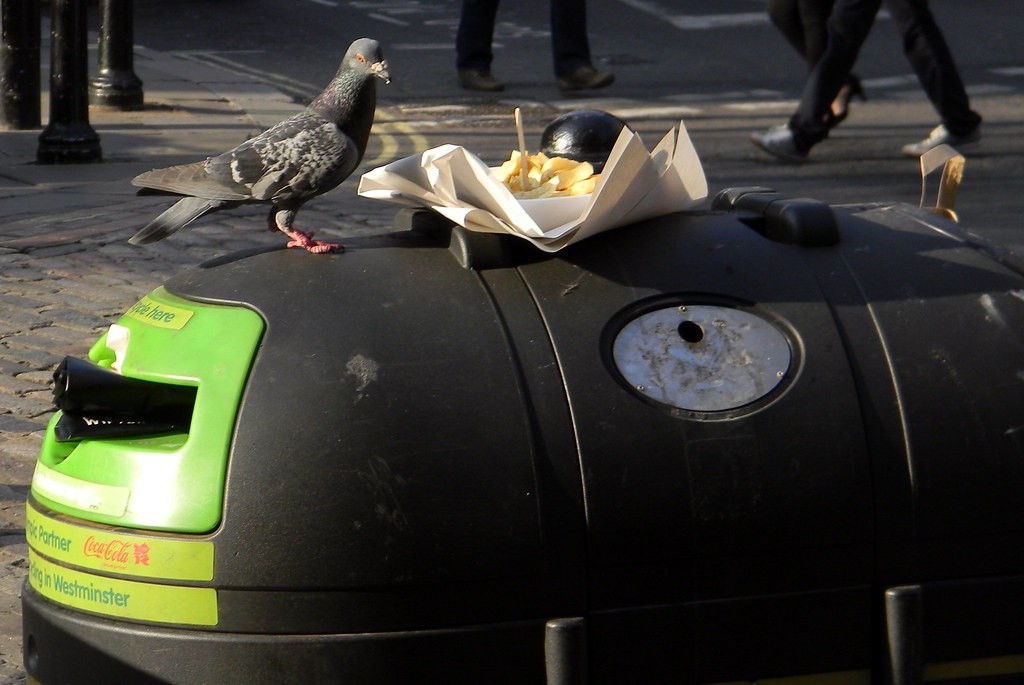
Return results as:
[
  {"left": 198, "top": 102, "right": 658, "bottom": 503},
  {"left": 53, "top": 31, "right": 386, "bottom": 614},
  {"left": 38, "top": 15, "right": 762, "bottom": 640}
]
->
[{"left": 29, "top": 107, "right": 1024, "bottom": 685}]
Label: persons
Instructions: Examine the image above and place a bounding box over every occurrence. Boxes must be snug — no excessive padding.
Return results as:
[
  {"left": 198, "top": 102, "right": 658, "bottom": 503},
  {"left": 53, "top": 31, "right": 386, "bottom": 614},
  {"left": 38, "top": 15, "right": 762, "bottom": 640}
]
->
[
  {"left": 750, "top": 0, "right": 982, "bottom": 165},
  {"left": 455, "top": 0, "right": 616, "bottom": 92}
]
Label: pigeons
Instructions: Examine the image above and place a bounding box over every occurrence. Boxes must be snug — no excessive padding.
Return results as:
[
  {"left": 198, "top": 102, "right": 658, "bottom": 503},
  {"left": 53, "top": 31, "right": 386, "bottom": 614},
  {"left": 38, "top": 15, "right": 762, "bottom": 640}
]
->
[{"left": 128, "top": 35, "right": 393, "bottom": 255}]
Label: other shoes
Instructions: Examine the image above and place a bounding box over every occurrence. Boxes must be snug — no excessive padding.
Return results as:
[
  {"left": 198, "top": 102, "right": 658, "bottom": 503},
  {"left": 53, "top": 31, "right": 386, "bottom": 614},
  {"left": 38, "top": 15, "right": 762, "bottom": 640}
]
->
[
  {"left": 902, "top": 123, "right": 982, "bottom": 158},
  {"left": 748, "top": 124, "right": 805, "bottom": 162},
  {"left": 456, "top": 63, "right": 505, "bottom": 91},
  {"left": 555, "top": 64, "right": 615, "bottom": 92}
]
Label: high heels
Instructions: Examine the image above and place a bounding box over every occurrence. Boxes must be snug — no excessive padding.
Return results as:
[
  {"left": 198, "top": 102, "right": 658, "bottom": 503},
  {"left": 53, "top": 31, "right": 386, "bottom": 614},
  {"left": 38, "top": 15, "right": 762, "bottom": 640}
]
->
[{"left": 822, "top": 74, "right": 868, "bottom": 130}]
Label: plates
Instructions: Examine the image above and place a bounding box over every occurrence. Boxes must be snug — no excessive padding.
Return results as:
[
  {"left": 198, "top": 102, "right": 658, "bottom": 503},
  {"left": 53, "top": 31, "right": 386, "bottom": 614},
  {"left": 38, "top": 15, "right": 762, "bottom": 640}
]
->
[{"left": 509, "top": 185, "right": 598, "bottom": 231}]
